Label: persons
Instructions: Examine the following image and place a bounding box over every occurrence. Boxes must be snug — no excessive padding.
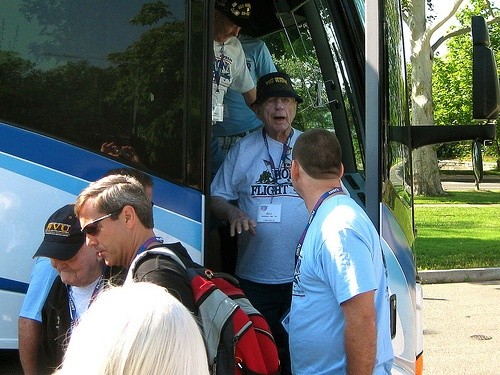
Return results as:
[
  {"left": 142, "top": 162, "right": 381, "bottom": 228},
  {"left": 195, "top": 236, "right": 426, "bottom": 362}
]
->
[
  {"left": 289, "top": 130, "right": 395, "bottom": 375},
  {"left": 17, "top": 0, "right": 351, "bottom": 375}
]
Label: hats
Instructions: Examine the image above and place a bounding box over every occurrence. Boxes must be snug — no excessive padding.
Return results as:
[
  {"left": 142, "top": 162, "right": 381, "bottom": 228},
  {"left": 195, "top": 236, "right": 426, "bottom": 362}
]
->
[
  {"left": 32, "top": 204, "right": 86, "bottom": 261},
  {"left": 257, "top": 72, "right": 302, "bottom": 105},
  {"left": 214, "top": 0, "right": 257, "bottom": 27}
]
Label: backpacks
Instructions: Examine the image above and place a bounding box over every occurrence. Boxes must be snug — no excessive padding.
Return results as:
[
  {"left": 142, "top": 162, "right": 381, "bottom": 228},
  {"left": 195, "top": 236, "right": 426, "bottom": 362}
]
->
[{"left": 123, "top": 247, "right": 281, "bottom": 375}]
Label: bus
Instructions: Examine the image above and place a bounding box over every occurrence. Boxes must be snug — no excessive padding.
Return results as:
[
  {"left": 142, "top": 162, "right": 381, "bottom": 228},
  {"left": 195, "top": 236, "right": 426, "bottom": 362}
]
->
[{"left": 1, "top": 1, "right": 499, "bottom": 375}]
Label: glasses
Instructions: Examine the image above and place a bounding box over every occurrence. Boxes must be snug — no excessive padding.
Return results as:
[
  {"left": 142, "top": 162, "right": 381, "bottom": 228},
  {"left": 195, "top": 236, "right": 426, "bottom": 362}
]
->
[
  {"left": 80, "top": 209, "right": 122, "bottom": 237},
  {"left": 268, "top": 97, "right": 296, "bottom": 104}
]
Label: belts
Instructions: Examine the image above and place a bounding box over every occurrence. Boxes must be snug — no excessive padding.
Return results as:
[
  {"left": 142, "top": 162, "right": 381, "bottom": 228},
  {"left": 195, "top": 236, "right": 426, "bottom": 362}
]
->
[{"left": 237, "top": 125, "right": 263, "bottom": 137}]
